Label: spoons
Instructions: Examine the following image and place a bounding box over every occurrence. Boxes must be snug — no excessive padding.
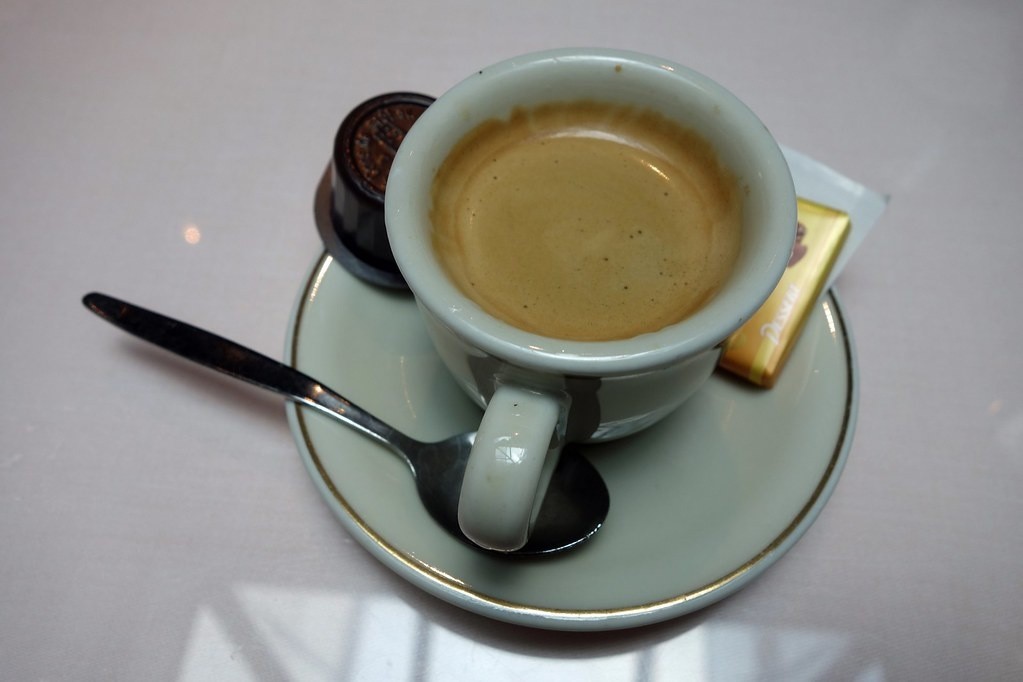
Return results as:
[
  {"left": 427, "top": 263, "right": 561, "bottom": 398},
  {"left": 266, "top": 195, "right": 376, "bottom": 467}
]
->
[{"left": 83, "top": 290, "right": 612, "bottom": 554}]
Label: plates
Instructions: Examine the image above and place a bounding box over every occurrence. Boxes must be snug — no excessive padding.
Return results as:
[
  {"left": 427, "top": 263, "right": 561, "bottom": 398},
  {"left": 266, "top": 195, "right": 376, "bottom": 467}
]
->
[{"left": 283, "top": 247, "right": 858, "bottom": 634}]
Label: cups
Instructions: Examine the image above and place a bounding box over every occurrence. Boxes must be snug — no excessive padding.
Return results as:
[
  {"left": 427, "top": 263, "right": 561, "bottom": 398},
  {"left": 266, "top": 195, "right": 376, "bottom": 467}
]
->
[{"left": 383, "top": 50, "right": 798, "bottom": 553}]
